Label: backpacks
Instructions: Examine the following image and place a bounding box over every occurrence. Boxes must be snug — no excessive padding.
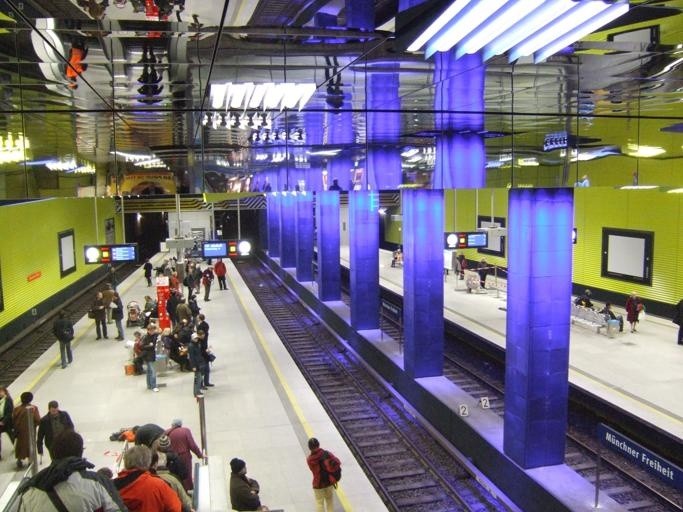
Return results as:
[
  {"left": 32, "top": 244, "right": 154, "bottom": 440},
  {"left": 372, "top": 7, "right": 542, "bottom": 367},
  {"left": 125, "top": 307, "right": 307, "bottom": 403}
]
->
[{"left": 318, "top": 452, "right": 341, "bottom": 488}]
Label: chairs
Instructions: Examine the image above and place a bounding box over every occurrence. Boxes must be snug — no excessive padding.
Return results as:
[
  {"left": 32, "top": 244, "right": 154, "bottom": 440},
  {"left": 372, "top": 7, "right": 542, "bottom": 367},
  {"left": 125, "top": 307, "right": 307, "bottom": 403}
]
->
[
  {"left": 571, "top": 302, "right": 610, "bottom": 334},
  {"left": 155, "top": 337, "right": 173, "bottom": 368},
  {"left": 464, "top": 270, "right": 507, "bottom": 293}
]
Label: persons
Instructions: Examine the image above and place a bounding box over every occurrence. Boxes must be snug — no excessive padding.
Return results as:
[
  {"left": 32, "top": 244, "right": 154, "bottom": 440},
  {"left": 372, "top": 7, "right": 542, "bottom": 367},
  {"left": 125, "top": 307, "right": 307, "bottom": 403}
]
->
[
  {"left": 152, "top": 257, "right": 229, "bottom": 301},
  {"left": 12, "top": 392, "right": 41, "bottom": 468},
  {"left": 132, "top": 286, "right": 215, "bottom": 399},
  {"left": 102, "top": 283, "right": 115, "bottom": 324},
  {"left": 391, "top": 248, "right": 402, "bottom": 268},
  {"left": 18, "top": 430, "right": 129, "bottom": 511},
  {"left": 599, "top": 302, "right": 623, "bottom": 332},
  {"left": 672, "top": 299, "right": 683, "bottom": 345},
  {"left": 62, "top": 1, "right": 185, "bottom": 105},
  {"left": 458, "top": 251, "right": 466, "bottom": 280},
  {"left": 111, "top": 292, "right": 125, "bottom": 342},
  {"left": 132, "top": 419, "right": 208, "bottom": 511},
  {"left": 144, "top": 259, "right": 153, "bottom": 287},
  {"left": 478, "top": 257, "right": 488, "bottom": 288},
  {"left": 307, "top": 438, "right": 341, "bottom": 512},
  {"left": 111, "top": 446, "right": 182, "bottom": 511},
  {"left": 230, "top": 458, "right": 270, "bottom": 512},
  {"left": 93, "top": 292, "right": 110, "bottom": 341},
  {"left": 626, "top": 292, "right": 639, "bottom": 333},
  {"left": 325, "top": 56, "right": 344, "bottom": 114},
  {"left": 53, "top": 308, "right": 74, "bottom": 369},
  {"left": 1, "top": 387, "right": 15, "bottom": 444},
  {"left": 37, "top": 400, "right": 74, "bottom": 462},
  {"left": 580, "top": 289, "right": 594, "bottom": 311}
]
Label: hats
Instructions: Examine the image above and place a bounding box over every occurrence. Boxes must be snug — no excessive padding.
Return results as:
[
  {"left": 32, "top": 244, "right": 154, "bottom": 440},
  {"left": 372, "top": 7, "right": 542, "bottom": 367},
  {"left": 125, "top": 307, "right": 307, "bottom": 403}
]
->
[
  {"left": 159, "top": 437, "right": 171, "bottom": 451},
  {"left": 230, "top": 459, "right": 245, "bottom": 472}
]
